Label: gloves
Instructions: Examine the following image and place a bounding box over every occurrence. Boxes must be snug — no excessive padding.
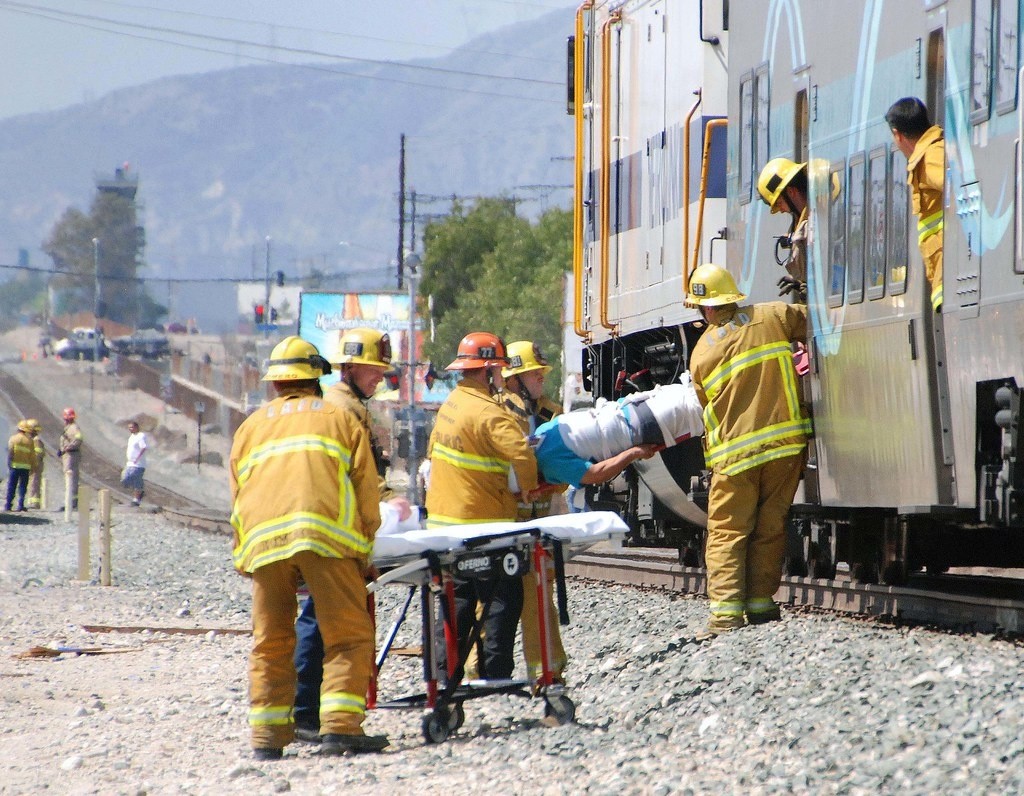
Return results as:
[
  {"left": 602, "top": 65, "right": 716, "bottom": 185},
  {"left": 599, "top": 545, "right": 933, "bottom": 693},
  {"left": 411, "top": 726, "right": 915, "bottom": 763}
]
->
[
  {"left": 57, "top": 450, "right": 62, "bottom": 456},
  {"left": 777, "top": 276, "right": 802, "bottom": 297}
]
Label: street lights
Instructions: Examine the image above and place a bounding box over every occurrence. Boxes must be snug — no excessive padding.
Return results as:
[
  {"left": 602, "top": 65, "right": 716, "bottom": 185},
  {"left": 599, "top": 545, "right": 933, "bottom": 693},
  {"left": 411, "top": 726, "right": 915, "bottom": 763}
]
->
[
  {"left": 262, "top": 234, "right": 274, "bottom": 337},
  {"left": 91, "top": 237, "right": 103, "bottom": 362},
  {"left": 400, "top": 251, "right": 422, "bottom": 503}
]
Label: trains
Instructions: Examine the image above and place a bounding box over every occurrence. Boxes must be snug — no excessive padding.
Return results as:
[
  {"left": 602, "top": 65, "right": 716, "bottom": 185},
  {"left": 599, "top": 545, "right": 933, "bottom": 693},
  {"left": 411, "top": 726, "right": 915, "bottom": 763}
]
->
[{"left": 558, "top": 1, "right": 1022, "bottom": 573}]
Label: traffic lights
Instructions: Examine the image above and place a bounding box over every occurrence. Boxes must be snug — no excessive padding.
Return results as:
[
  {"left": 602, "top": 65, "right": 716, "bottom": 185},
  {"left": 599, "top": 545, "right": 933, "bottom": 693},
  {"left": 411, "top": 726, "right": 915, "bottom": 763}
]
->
[
  {"left": 254, "top": 305, "right": 264, "bottom": 323},
  {"left": 386, "top": 372, "right": 401, "bottom": 390}
]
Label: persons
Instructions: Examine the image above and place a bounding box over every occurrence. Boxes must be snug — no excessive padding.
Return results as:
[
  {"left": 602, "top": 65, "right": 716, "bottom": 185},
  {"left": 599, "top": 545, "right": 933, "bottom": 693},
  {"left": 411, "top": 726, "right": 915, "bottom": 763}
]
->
[
  {"left": 56, "top": 408, "right": 83, "bottom": 511},
  {"left": 463, "top": 339, "right": 587, "bottom": 697},
  {"left": 423, "top": 331, "right": 542, "bottom": 697},
  {"left": 885, "top": 97, "right": 944, "bottom": 313},
  {"left": 682, "top": 263, "right": 809, "bottom": 630},
  {"left": 4, "top": 418, "right": 45, "bottom": 512},
  {"left": 120, "top": 421, "right": 148, "bottom": 507},
  {"left": 758, "top": 157, "right": 809, "bottom": 305},
  {"left": 229, "top": 336, "right": 390, "bottom": 760},
  {"left": 293, "top": 327, "right": 392, "bottom": 742},
  {"left": 509, "top": 371, "right": 714, "bottom": 488}
]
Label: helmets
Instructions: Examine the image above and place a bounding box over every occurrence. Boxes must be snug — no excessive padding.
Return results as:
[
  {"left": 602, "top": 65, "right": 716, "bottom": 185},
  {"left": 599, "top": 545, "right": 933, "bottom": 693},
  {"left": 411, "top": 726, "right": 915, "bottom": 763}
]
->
[
  {"left": 63, "top": 408, "right": 75, "bottom": 419},
  {"left": 27, "top": 419, "right": 42, "bottom": 430},
  {"left": 261, "top": 336, "right": 323, "bottom": 380},
  {"left": 757, "top": 158, "right": 808, "bottom": 214},
  {"left": 685, "top": 263, "right": 747, "bottom": 306},
  {"left": 17, "top": 420, "right": 30, "bottom": 431},
  {"left": 328, "top": 329, "right": 397, "bottom": 371},
  {"left": 500, "top": 340, "right": 553, "bottom": 378},
  {"left": 444, "top": 332, "right": 513, "bottom": 370}
]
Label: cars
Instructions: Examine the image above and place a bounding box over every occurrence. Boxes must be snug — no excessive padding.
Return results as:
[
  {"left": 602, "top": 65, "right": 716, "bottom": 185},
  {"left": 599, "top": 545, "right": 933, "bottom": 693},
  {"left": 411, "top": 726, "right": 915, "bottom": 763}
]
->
[
  {"left": 110, "top": 325, "right": 171, "bottom": 358},
  {"left": 54, "top": 328, "right": 110, "bottom": 359}
]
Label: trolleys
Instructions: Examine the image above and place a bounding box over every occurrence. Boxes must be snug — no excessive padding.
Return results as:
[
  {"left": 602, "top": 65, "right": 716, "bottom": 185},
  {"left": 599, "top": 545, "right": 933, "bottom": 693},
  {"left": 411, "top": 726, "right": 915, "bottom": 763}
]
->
[{"left": 295, "top": 510, "right": 630, "bottom": 745}]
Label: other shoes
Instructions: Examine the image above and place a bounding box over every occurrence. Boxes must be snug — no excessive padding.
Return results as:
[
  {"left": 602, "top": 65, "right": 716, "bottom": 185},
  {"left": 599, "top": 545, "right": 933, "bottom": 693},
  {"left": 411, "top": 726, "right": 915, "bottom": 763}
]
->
[
  {"left": 294, "top": 726, "right": 324, "bottom": 745},
  {"left": 129, "top": 502, "right": 139, "bottom": 508},
  {"left": 746, "top": 607, "right": 781, "bottom": 624},
  {"left": 321, "top": 732, "right": 390, "bottom": 754},
  {"left": 695, "top": 625, "right": 733, "bottom": 641},
  {"left": 254, "top": 748, "right": 283, "bottom": 759}
]
